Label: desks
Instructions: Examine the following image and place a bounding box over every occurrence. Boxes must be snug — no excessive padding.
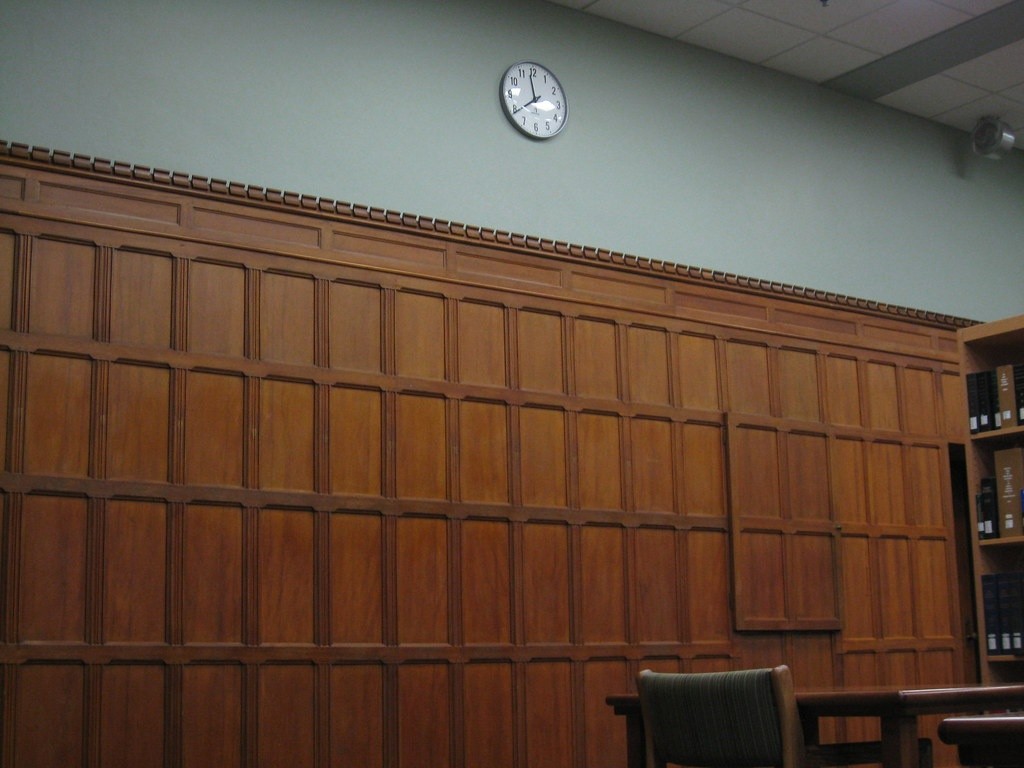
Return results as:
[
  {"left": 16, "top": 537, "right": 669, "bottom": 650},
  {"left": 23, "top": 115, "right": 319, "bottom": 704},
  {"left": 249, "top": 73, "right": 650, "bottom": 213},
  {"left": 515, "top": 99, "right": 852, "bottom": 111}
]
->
[{"left": 605, "top": 683, "right": 1024, "bottom": 768}]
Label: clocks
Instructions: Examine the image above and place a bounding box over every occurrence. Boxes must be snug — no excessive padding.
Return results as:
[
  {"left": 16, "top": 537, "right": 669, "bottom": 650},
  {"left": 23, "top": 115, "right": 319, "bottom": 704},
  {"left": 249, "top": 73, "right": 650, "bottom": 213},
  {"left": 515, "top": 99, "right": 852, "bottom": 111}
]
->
[{"left": 499, "top": 59, "right": 569, "bottom": 142}]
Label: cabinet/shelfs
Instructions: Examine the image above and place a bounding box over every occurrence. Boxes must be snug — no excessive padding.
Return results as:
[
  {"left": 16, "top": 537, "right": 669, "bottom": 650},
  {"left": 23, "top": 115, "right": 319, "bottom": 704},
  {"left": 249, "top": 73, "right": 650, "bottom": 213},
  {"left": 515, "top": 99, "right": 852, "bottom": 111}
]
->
[{"left": 956, "top": 313, "right": 1024, "bottom": 716}]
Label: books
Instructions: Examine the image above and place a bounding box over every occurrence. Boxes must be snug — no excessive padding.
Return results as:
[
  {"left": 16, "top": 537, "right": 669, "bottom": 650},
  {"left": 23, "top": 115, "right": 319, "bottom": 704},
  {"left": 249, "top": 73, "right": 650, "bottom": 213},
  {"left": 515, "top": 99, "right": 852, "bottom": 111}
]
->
[
  {"left": 966, "top": 363, "right": 1024, "bottom": 434},
  {"left": 975, "top": 445, "right": 1024, "bottom": 539},
  {"left": 982, "top": 572, "right": 1024, "bottom": 659}
]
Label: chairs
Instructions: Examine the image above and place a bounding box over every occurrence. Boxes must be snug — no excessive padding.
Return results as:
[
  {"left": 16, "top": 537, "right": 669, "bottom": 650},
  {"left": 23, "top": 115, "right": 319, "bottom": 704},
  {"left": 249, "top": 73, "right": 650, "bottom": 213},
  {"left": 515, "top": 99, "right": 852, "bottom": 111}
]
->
[
  {"left": 635, "top": 665, "right": 934, "bottom": 768},
  {"left": 937, "top": 715, "right": 1024, "bottom": 768}
]
